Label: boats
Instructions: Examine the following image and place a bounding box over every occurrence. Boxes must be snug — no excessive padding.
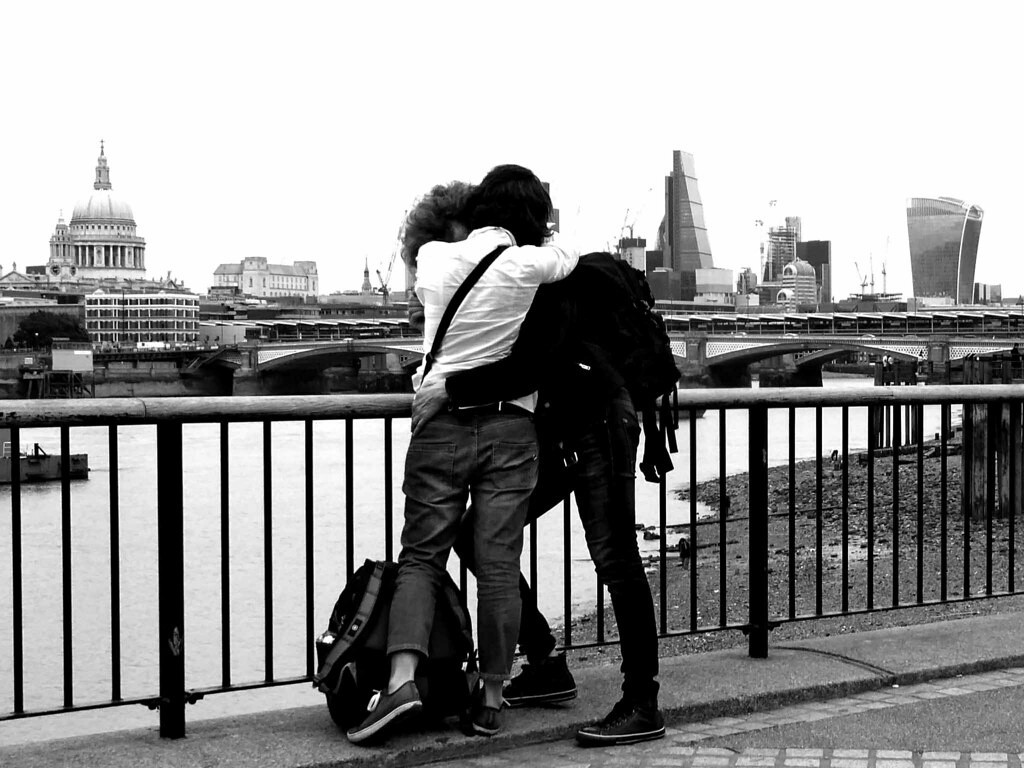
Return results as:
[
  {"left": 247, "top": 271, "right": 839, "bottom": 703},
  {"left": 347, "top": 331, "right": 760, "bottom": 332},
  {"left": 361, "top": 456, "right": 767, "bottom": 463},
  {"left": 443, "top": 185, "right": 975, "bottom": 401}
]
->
[{"left": 0, "top": 427, "right": 90, "bottom": 484}]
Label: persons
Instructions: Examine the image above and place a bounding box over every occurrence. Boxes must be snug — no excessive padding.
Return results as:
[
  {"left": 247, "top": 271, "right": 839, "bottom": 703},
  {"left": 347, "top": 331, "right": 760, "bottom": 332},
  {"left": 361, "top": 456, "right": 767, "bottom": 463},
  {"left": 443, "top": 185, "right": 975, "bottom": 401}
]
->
[
  {"left": 345, "top": 165, "right": 580, "bottom": 740},
  {"left": 401, "top": 182, "right": 666, "bottom": 746}
]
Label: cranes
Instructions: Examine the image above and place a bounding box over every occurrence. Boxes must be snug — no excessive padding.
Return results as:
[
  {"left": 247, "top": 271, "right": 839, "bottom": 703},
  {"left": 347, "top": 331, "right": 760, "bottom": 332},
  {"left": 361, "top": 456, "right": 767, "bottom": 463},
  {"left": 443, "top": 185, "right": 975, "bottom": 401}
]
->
[
  {"left": 881, "top": 235, "right": 889, "bottom": 294},
  {"left": 378, "top": 196, "right": 413, "bottom": 302},
  {"left": 855, "top": 262, "right": 868, "bottom": 294},
  {"left": 869, "top": 253, "right": 875, "bottom": 294}
]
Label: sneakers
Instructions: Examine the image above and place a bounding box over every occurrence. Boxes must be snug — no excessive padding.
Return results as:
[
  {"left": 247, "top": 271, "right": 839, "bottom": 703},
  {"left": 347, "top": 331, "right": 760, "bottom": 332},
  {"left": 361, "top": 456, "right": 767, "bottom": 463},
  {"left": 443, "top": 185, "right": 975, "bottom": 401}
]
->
[
  {"left": 505, "top": 651, "right": 578, "bottom": 707},
  {"left": 576, "top": 698, "right": 666, "bottom": 743},
  {"left": 348, "top": 681, "right": 422, "bottom": 740},
  {"left": 474, "top": 699, "right": 503, "bottom": 734}
]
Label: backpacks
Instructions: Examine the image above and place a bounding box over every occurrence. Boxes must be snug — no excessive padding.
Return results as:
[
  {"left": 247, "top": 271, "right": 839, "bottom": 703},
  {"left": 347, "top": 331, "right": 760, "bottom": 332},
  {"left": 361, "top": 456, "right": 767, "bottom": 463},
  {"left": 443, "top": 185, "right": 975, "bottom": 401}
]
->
[
  {"left": 317, "top": 558, "right": 474, "bottom": 735},
  {"left": 579, "top": 252, "right": 683, "bottom": 481}
]
